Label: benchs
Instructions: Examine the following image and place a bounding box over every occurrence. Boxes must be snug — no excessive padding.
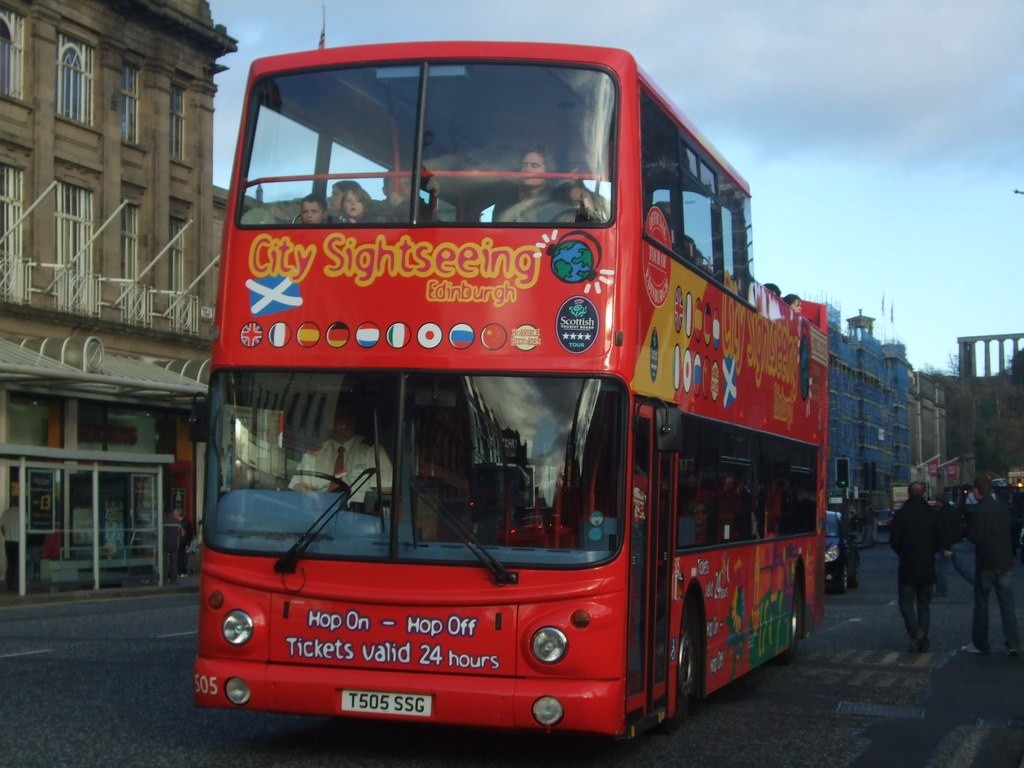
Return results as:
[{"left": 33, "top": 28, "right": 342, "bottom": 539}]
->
[{"left": 48, "top": 543, "right": 159, "bottom": 592}]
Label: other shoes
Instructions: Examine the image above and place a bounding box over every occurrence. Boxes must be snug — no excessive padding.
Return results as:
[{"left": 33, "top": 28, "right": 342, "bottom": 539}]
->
[
  {"left": 909, "top": 628, "right": 924, "bottom": 652},
  {"left": 920, "top": 638, "right": 929, "bottom": 653},
  {"left": 179, "top": 573, "right": 188, "bottom": 578},
  {"left": 1004, "top": 647, "right": 1019, "bottom": 656},
  {"left": 932, "top": 591, "right": 946, "bottom": 597},
  {"left": 961, "top": 642, "right": 985, "bottom": 654}
]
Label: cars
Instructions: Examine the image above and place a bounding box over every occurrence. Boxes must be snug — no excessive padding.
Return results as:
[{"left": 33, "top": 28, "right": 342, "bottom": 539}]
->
[
  {"left": 824, "top": 510, "right": 862, "bottom": 594},
  {"left": 873, "top": 508, "right": 895, "bottom": 527}
]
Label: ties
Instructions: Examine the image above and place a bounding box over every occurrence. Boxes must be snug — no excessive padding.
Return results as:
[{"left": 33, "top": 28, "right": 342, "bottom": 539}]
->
[{"left": 328, "top": 446, "right": 345, "bottom": 491}]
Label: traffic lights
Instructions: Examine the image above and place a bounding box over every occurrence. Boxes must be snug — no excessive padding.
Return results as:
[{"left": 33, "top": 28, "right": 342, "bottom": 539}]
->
[{"left": 834, "top": 455, "right": 851, "bottom": 491}]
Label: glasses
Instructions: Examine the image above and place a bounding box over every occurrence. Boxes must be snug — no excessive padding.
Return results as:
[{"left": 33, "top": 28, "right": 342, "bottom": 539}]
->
[{"left": 693, "top": 509, "right": 706, "bottom": 514}]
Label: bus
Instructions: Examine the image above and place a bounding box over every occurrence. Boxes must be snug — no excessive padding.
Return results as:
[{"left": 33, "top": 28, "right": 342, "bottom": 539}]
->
[{"left": 179, "top": 39, "right": 832, "bottom": 740}]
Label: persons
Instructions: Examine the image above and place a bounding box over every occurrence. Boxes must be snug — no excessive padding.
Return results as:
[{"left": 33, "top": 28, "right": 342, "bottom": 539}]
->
[
  {"left": 163, "top": 507, "right": 179, "bottom": 586},
  {"left": 301, "top": 168, "right": 442, "bottom": 224},
  {"left": 492, "top": 145, "right": 611, "bottom": 222},
  {"left": 172, "top": 509, "right": 192, "bottom": 578},
  {"left": 0, "top": 495, "right": 29, "bottom": 590},
  {"left": 931, "top": 492, "right": 975, "bottom": 598},
  {"left": 783, "top": 294, "right": 802, "bottom": 313},
  {"left": 288, "top": 404, "right": 393, "bottom": 509},
  {"left": 961, "top": 477, "right": 1018, "bottom": 656},
  {"left": 889, "top": 481, "right": 953, "bottom": 652},
  {"left": 763, "top": 283, "right": 781, "bottom": 297}
]
